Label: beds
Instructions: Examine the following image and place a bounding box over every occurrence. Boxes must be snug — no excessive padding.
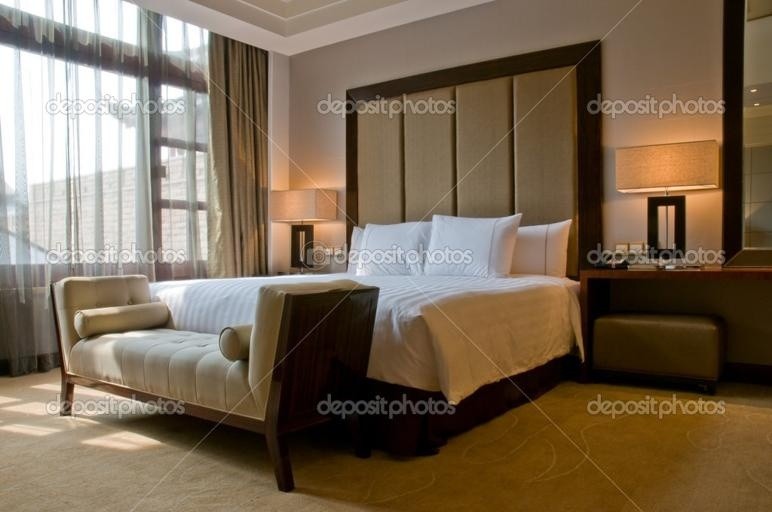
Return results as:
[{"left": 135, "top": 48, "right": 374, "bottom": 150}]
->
[{"left": 124, "top": 274, "right": 579, "bottom": 456}]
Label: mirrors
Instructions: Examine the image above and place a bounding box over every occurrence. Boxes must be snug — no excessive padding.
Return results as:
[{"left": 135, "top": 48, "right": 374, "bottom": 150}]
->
[{"left": 724, "top": 1, "right": 771, "bottom": 265}]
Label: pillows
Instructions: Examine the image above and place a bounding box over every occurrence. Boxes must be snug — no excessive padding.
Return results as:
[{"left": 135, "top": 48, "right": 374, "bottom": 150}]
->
[{"left": 341, "top": 212, "right": 572, "bottom": 282}]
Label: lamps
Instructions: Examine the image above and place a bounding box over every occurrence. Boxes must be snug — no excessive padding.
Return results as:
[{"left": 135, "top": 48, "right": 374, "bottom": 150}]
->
[
  {"left": 612, "top": 139, "right": 722, "bottom": 256},
  {"left": 268, "top": 190, "right": 337, "bottom": 270}
]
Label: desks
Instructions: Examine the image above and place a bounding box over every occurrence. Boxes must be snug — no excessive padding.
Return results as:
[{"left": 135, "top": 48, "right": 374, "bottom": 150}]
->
[{"left": 580, "top": 264, "right": 770, "bottom": 380}]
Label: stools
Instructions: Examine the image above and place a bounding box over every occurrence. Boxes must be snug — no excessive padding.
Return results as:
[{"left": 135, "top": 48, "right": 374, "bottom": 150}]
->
[{"left": 594, "top": 314, "right": 722, "bottom": 394}]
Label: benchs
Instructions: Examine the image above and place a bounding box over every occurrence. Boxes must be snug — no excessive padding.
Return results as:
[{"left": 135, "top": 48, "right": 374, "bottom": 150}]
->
[{"left": 46, "top": 274, "right": 379, "bottom": 493}]
